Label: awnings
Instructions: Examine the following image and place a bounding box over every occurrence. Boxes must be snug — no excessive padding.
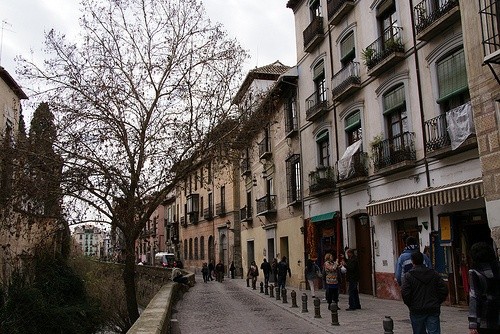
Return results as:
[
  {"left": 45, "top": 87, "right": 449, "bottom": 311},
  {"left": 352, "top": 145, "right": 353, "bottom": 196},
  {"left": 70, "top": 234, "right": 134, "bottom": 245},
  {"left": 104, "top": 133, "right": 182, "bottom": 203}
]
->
[{"left": 366, "top": 177, "right": 484, "bottom": 217}]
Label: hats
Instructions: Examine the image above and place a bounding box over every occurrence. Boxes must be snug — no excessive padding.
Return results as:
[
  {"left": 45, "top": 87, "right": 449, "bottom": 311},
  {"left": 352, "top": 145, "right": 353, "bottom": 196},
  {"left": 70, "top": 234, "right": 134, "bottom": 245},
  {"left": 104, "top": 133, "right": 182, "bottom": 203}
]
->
[{"left": 405, "top": 236, "right": 417, "bottom": 244}]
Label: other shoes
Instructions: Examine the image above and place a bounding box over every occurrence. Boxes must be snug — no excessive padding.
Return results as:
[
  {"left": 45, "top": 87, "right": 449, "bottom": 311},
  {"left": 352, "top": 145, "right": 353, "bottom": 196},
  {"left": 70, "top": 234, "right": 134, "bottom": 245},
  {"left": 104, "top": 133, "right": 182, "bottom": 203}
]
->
[{"left": 346, "top": 306, "right": 361, "bottom": 311}]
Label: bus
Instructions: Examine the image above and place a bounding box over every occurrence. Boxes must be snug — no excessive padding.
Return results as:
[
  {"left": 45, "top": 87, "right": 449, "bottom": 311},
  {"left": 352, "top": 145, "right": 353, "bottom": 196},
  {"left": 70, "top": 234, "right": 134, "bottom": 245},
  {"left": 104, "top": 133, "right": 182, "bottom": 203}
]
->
[{"left": 155, "top": 252, "right": 175, "bottom": 268}]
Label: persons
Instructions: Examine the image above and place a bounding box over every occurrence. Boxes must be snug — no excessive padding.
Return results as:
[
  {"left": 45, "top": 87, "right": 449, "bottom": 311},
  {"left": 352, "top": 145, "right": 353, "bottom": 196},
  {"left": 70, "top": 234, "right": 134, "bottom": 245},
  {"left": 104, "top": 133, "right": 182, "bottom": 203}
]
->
[
  {"left": 271, "top": 257, "right": 279, "bottom": 287},
  {"left": 246, "top": 261, "right": 259, "bottom": 288},
  {"left": 400, "top": 251, "right": 450, "bottom": 334},
  {"left": 173, "top": 259, "right": 184, "bottom": 269},
  {"left": 323, "top": 253, "right": 341, "bottom": 311},
  {"left": 138, "top": 259, "right": 147, "bottom": 265},
  {"left": 277, "top": 256, "right": 291, "bottom": 290},
  {"left": 261, "top": 258, "right": 272, "bottom": 287},
  {"left": 393, "top": 237, "right": 431, "bottom": 288},
  {"left": 466, "top": 241, "right": 500, "bottom": 334},
  {"left": 201, "top": 260, "right": 225, "bottom": 284},
  {"left": 341, "top": 247, "right": 362, "bottom": 311},
  {"left": 335, "top": 249, "right": 348, "bottom": 293},
  {"left": 305, "top": 254, "right": 320, "bottom": 298},
  {"left": 229, "top": 261, "right": 236, "bottom": 279}
]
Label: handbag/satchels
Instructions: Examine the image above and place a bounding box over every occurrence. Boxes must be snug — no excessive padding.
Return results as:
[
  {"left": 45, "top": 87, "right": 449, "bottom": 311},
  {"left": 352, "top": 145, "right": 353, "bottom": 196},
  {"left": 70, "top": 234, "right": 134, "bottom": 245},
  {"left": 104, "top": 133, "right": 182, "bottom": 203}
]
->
[
  {"left": 317, "top": 268, "right": 322, "bottom": 277},
  {"left": 341, "top": 264, "right": 347, "bottom": 274}
]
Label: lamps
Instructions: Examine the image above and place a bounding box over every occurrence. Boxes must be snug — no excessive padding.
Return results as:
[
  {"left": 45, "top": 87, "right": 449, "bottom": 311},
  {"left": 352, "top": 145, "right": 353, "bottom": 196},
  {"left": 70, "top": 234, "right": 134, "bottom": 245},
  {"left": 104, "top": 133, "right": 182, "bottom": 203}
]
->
[
  {"left": 253, "top": 173, "right": 261, "bottom": 186},
  {"left": 359, "top": 216, "right": 375, "bottom": 234},
  {"left": 226, "top": 220, "right": 236, "bottom": 232}
]
